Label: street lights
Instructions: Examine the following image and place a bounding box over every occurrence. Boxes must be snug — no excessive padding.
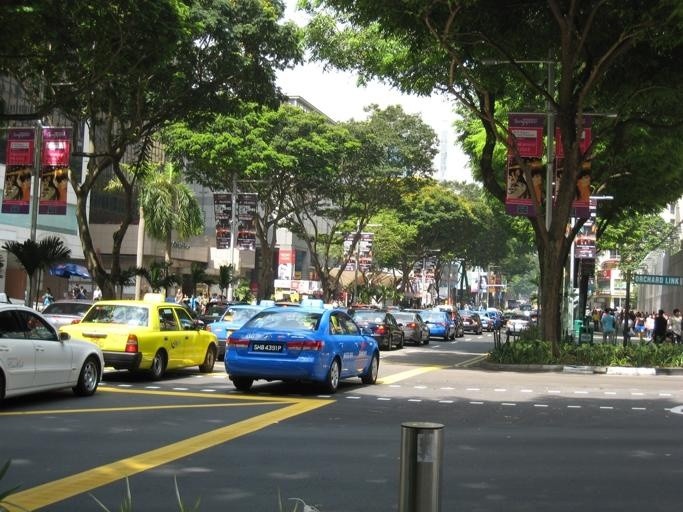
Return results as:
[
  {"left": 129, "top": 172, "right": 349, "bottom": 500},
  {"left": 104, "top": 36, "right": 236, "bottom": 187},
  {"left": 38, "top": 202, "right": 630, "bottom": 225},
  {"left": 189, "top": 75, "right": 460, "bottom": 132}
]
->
[
  {"left": 227, "top": 171, "right": 280, "bottom": 302},
  {"left": 484, "top": 262, "right": 502, "bottom": 310},
  {"left": 453, "top": 51, "right": 553, "bottom": 244},
  {"left": 420, "top": 247, "right": 444, "bottom": 308}
]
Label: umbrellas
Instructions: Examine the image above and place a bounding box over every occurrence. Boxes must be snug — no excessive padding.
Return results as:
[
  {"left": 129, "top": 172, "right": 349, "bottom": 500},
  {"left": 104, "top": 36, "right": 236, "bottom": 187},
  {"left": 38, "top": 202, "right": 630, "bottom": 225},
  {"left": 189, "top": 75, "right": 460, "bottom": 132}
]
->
[{"left": 47, "top": 261, "right": 91, "bottom": 292}]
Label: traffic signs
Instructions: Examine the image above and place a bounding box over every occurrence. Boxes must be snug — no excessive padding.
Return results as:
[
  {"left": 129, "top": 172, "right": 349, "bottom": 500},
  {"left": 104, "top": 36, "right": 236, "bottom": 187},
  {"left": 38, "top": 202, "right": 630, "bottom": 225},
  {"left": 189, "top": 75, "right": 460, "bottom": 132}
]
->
[{"left": 633, "top": 274, "right": 683, "bottom": 286}]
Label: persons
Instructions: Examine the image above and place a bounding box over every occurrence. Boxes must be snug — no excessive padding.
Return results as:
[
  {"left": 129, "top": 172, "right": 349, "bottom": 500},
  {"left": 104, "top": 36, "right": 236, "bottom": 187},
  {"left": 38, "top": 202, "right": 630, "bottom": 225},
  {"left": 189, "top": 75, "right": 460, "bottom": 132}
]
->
[
  {"left": 79, "top": 286, "right": 86, "bottom": 299},
  {"left": 392, "top": 295, "right": 502, "bottom": 311},
  {"left": 2, "top": 165, "right": 31, "bottom": 202},
  {"left": 41, "top": 287, "right": 54, "bottom": 310},
  {"left": 40, "top": 169, "right": 66, "bottom": 201},
  {"left": 173, "top": 287, "right": 240, "bottom": 314},
  {"left": 589, "top": 305, "right": 683, "bottom": 347},
  {"left": 72, "top": 282, "right": 80, "bottom": 298},
  {"left": 480, "top": 275, "right": 488, "bottom": 293},
  {"left": 506, "top": 155, "right": 540, "bottom": 200},
  {"left": 92, "top": 286, "right": 102, "bottom": 300}
]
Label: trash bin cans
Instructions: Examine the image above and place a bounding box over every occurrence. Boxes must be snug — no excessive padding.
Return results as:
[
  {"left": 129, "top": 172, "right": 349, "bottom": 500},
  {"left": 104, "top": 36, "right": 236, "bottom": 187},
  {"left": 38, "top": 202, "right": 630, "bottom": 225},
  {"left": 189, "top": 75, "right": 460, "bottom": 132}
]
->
[
  {"left": 579, "top": 326, "right": 594, "bottom": 347},
  {"left": 574, "top": 319, "right": 583, "bottom": 337}
]
